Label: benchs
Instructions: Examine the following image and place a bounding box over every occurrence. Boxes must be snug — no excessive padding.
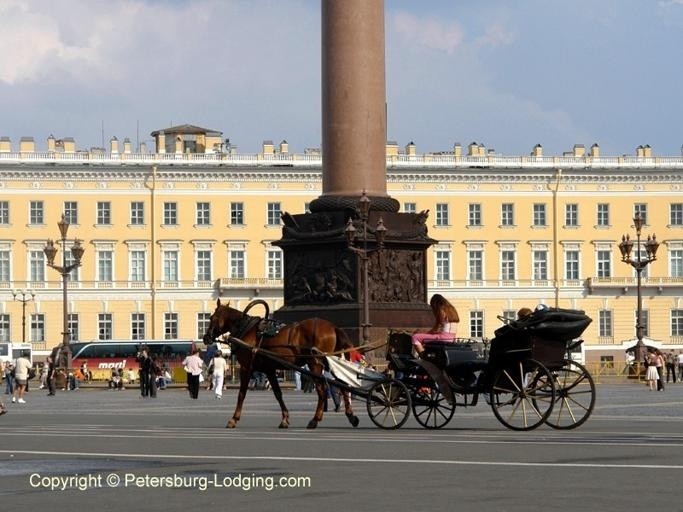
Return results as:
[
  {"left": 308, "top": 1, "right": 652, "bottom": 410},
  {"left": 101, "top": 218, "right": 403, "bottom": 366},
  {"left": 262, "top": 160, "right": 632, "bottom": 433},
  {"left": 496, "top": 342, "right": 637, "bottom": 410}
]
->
[{"left": 421, "top": 339, "right": 471, "bottom": 367}]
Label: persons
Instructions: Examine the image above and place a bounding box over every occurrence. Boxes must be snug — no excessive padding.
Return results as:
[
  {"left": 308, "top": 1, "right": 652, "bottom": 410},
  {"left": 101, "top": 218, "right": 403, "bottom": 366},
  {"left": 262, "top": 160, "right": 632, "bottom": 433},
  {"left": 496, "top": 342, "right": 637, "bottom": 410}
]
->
[
  {"left": 518, "top": 308, "right": 533, "bottom": 320},
  {"left": 625, "top": 349, "right": 683, "bottom": 391},
  {"left": 412, "top": 294, "right": 459, "bottom": 359},
  {"left": 0, "top": 349, "right": 226, "bottom": 414},
  {"left": 292, "top": 364, "right": 433, "bottom": 399}
]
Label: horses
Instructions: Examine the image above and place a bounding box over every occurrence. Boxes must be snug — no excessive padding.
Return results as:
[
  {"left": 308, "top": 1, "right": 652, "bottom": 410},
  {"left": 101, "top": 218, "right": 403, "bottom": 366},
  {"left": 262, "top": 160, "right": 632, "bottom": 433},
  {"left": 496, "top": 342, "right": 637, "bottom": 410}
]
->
[{"left": 203, "top": 298, "right": 360, "bottom": 429}]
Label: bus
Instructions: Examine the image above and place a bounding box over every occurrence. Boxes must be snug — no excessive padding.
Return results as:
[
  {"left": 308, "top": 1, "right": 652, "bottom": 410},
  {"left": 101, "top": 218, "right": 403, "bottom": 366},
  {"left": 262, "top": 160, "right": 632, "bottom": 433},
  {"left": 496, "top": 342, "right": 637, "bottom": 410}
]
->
[{"left": 49, "top": 340, "right": 195, "bottom": 383}]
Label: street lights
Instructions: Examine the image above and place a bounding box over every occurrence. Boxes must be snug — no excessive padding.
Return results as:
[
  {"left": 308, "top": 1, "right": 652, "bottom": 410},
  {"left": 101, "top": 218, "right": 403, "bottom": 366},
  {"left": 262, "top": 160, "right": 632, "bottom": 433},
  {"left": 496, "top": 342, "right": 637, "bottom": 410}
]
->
[
  {"left": 618, "top": 209, "right": 660, "bottom": 381},
  {"left": 12, "top": 290, "right": 36, "bottom": 343},
  {"left": 43, "top": 212, "right": 84, "bottom": 390}
]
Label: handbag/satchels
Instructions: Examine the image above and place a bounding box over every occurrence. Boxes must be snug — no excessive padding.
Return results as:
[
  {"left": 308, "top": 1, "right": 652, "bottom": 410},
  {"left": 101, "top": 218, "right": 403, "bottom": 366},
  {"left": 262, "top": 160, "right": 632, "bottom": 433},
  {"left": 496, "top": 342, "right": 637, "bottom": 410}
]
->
[{"left": 209, "top": 365, "right": 214, "bottom": 374}]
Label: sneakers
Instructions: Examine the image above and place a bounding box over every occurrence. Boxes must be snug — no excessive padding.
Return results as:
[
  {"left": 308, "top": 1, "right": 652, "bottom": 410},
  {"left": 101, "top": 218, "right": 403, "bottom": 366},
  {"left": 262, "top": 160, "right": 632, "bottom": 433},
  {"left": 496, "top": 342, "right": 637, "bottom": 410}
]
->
[
  {"left": 12, "top": 397, "right": 15, "bottom": 402},
  {"left": 18, "top": 398, "right": 26, "bottom": 403}
]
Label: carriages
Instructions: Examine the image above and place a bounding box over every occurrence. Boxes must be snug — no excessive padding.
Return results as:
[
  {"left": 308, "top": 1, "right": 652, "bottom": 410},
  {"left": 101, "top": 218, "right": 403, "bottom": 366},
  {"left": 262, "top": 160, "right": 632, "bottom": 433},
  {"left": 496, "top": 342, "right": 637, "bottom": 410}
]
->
[{"left": 202, "top": 297, "right": 597, "bottom": 428}]
